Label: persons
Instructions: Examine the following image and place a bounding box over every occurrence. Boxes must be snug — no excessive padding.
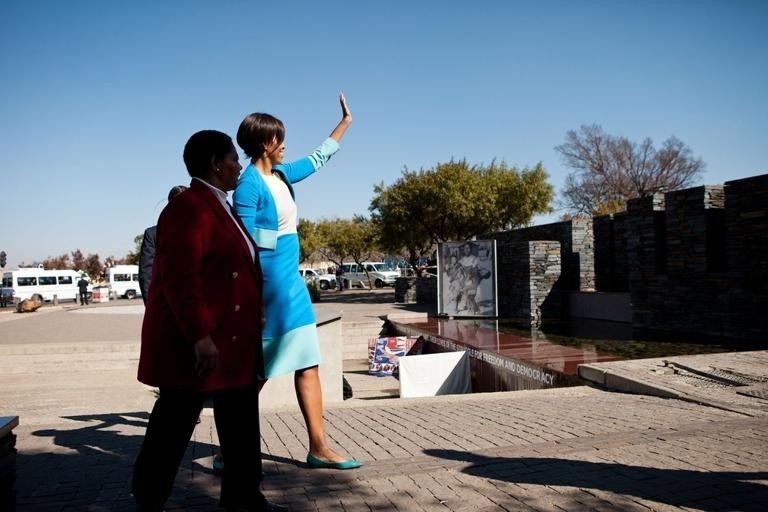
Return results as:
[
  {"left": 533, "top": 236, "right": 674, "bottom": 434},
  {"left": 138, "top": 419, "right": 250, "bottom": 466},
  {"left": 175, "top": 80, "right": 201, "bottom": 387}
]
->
[
  {"left": 136, "top": 184, "right": 193, "bottom": 305},
  {"left": 444, "top": 253, "right": 467, "bottom": 312},
  {"left": 77, "top": 275, "right": 91, "bottom": 306},
  {"left": 212, "top": 90, "right": 364, "bottom": 478},
  {"left": 457, "top": 243, "right": 481, "bottom": 311},
  {"left": 458, "top": 266, "right": 492, "bottom": 314},
  {"left": 129, "top": 128, "right": 290, "bottom": 511}
]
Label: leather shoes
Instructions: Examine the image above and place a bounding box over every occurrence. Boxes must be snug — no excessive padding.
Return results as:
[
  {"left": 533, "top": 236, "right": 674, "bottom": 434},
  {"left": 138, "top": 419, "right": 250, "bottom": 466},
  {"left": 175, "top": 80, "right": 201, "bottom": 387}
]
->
[
  {"left": 260, "top": 498, "right": 287, "bottom": 511},
  {"left": 306, "top": 452, "right": 362, "bottom": 469},
  {"left": 212, "top": 456, "right": 269, "bottom": 478}
]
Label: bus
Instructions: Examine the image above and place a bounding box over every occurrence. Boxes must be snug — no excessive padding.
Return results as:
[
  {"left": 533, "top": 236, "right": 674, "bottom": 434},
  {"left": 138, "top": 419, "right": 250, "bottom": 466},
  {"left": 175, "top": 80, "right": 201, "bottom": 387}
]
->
[
  {"left": 1, "top": 270, "right": 95, "bottom": 304},
  {"left": 338, "top": 262, "right": 401, "bottom": 287},
  {"left": 105, "top": 265, "right": 143, "bottom": 301}
]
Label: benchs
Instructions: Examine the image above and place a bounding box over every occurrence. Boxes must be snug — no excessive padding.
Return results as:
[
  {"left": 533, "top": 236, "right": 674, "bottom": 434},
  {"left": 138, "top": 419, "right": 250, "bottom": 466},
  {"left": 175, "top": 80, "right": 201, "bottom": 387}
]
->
[{"left": 0, "top": 415, "right": 20, "bottom": 482}]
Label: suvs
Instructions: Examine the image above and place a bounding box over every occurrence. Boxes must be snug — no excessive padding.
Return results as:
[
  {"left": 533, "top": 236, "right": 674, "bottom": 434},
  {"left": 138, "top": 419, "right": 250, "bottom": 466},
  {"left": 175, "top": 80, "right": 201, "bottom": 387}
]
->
[{"left": 300, "top": 267, "right": 337, "bottom": 290}]
